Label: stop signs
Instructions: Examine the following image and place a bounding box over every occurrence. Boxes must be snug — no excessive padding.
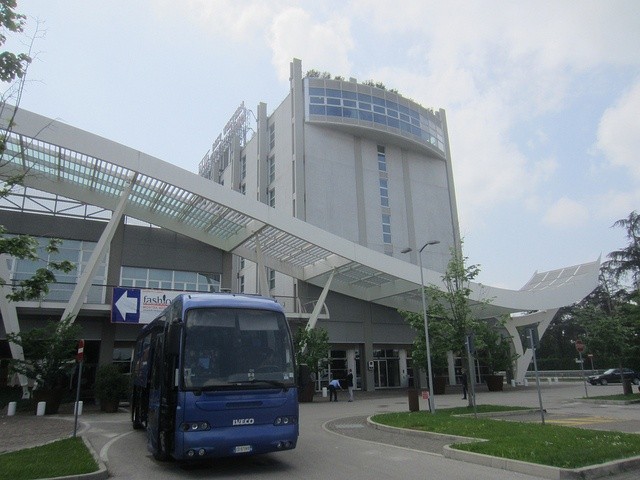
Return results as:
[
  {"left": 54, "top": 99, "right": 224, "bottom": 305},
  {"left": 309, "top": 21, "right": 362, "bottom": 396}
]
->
[{"left": 77, "top": 339, "right": 84, "bottom": 362}]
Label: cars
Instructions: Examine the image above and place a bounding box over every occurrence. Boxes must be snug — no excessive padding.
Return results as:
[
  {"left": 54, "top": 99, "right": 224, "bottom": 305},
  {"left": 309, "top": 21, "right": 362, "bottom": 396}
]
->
[{"left": 586, "top": 367, "right": 636, "bottom": 386}]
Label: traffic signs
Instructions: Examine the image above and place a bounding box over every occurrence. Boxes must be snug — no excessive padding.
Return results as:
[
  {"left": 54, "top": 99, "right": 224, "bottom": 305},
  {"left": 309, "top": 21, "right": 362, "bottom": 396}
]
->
[{"left": 112, "top": 285, "right": 180, "bottom": 327}]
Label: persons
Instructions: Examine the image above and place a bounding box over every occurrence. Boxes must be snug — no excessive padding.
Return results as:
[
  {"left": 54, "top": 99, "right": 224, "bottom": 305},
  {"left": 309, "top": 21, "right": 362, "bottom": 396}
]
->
[
  {"left": 341, "top": 370, "right": 354, "bottom": 403},
  {"left": 459, "top": 369, "right": 470, "bottom": 401},
  {"left": 330, "top": 378, "right": 344, "bottom": 401}
]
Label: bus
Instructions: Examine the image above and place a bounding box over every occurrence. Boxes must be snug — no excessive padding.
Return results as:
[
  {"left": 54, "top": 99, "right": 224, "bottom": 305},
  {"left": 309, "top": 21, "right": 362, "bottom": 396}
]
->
[{"left": 131, "top": 293, "right": 298, "bottom": 461}]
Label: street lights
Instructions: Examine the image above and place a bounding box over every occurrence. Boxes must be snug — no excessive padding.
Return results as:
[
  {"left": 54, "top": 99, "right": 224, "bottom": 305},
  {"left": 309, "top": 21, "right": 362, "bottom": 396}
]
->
[{"left": 401, "top": 240, "right": 441, "bottom": 414}]
